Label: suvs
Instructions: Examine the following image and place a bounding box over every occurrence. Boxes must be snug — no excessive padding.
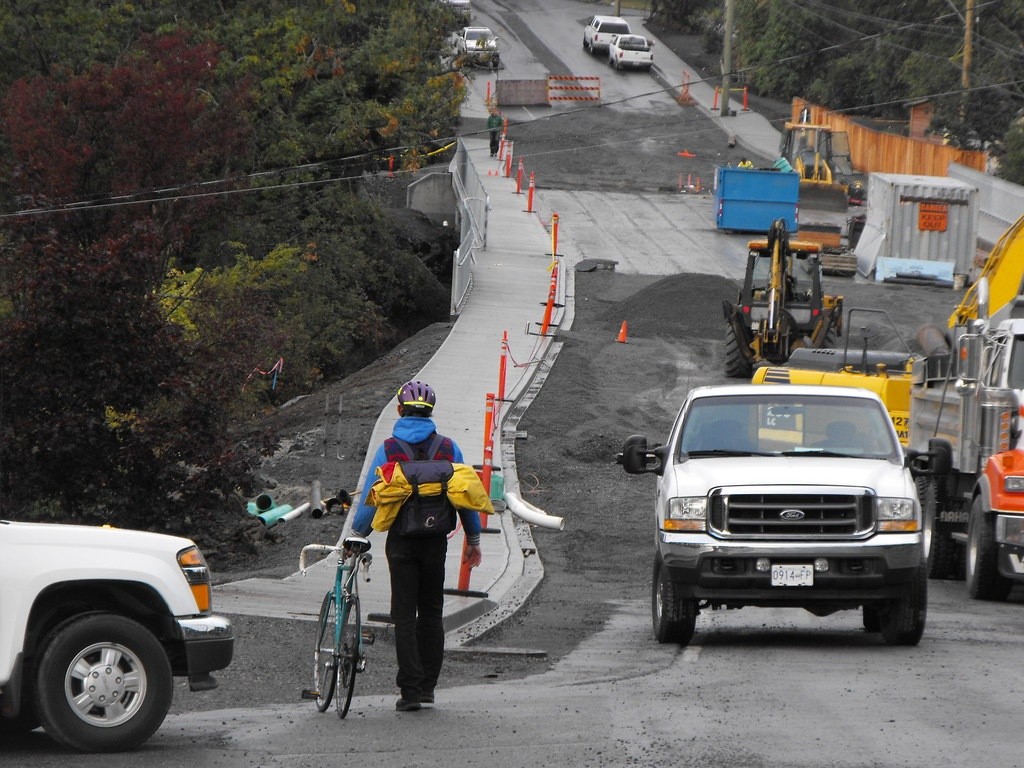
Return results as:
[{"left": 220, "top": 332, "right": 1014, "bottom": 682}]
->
[
  {"left": 622, "top": 385, "right": 927, "bottom": 647},
  {"left": 582, "top": 14, "right": 632, "bottom": 56}
]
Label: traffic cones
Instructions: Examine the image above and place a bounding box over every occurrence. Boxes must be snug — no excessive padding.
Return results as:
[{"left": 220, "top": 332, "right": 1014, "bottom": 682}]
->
[
  {"left": 488, "top": 170, "right": 498, "bottom": 176},
  {"left": 614, "top": 321, "right": 630, "bottom": 344}
]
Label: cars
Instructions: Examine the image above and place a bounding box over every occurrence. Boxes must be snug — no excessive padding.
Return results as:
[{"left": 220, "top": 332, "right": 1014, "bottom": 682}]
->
[
  {"left": 1, "top": 519, "right": 237, "bottom": 753},
  {"left": 442, "top": 0, "right": 471, "bottom": 14}
]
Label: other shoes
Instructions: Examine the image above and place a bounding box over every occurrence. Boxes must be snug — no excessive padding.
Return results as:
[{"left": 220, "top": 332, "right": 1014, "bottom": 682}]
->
[
  {"left": 422, "top": 698, "right": 435, "bottom": 708},
  {"left": 397, "top": 699, "right": 420, "bottom": 711}
]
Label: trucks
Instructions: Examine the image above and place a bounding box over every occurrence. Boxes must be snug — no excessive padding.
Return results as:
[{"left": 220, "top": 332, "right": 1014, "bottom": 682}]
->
[{"left": 909, "top": 277, "right": 1024, "bottom": 600}]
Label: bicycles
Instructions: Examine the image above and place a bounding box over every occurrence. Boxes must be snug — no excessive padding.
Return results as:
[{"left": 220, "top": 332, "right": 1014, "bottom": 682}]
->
[{"left": 297, "top": 537, "right": 380, "bottom": 719}]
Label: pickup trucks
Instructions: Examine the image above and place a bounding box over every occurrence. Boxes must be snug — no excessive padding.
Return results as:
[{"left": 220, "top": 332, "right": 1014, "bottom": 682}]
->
[
  {"left": 608, "top": 33, "right": 654, "bottom": 72},
  {"left": 456, "top": 25, "right": 499, "bottom": 65}
]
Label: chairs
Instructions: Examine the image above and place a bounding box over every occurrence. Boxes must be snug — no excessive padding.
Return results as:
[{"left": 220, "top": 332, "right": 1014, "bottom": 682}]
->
[
  {"left": 811, "top": 422, "right": 869, "bottom": 454},
  {"left": 703, "top": 420, "right": 758, "bottom": 452}
]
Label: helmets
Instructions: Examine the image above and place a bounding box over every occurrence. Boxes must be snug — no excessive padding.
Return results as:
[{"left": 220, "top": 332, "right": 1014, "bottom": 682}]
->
[{"left": 397, "top": 381, "right": 436, "bottom": 412}]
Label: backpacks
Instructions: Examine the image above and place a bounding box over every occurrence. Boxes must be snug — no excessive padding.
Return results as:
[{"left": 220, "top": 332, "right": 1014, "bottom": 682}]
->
[{"left": 390, "top": 435, "right": 452, "bottom": 538}]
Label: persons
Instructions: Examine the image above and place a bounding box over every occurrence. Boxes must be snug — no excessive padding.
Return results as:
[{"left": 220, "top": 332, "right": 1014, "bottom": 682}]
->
[
  {"left": 342, "top": 381, "right": 481, "bottom": 711},
  {"left": 943, "top": 128, "right": 950, "bottom": 143},
  {"left": 738, "top": 158, "right": 752, "bottom": 167},
  {"left": 488, "top": 111, "right": 504, "bottom": 156}
]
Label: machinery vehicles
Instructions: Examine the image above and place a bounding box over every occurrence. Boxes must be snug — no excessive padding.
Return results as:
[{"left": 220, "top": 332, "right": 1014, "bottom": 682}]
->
[
  {"left": 724, "top": 217, "right": 841, "bottom": 378},
  {"left": 776, "top": 123, "right": 851, "bottom": 213},
  {"left": 752, "top": 215, "right": 1024, "bottom": 459}
]
